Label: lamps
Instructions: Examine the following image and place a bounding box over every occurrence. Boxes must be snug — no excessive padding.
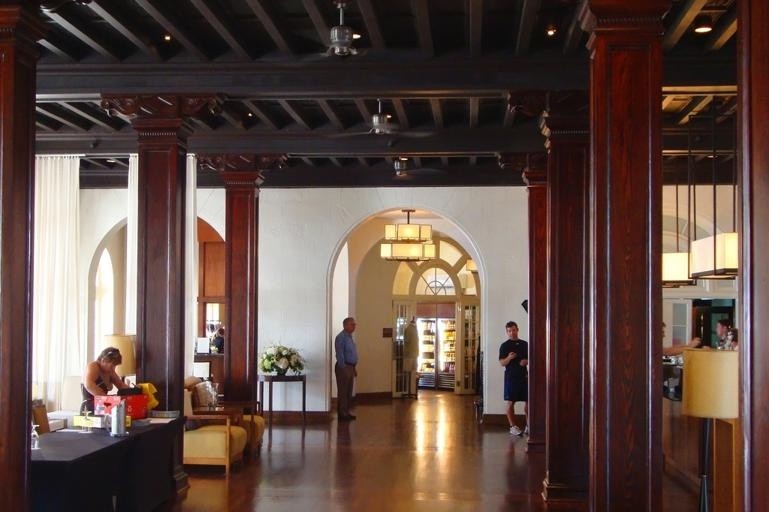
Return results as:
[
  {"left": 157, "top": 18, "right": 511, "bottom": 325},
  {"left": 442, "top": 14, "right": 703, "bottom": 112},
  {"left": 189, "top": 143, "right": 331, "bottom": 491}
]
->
[
  {"left": 378, "top": 205, "right": 438, "bottom": 264},
  {"left": 659, "top": 112, "right": 739, "bottom": 291},
  {"left": 99, "top": 334, "right": 137, "bottom": 384},
  {"left": 678, "top": 347, "right": 741, "bottom": 511}
]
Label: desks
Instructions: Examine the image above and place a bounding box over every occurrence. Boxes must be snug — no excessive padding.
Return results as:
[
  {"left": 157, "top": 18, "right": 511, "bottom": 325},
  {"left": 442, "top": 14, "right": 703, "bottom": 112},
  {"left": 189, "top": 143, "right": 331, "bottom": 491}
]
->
[{"left": 258, "top": 376, "right": 309, "bottom": 422}]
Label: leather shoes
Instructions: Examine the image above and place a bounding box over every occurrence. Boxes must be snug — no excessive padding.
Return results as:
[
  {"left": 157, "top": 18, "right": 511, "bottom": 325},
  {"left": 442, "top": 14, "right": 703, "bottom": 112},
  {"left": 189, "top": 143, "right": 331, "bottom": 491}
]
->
[{"left": 338, "top": 414, "right": 356, "bottom": 420}]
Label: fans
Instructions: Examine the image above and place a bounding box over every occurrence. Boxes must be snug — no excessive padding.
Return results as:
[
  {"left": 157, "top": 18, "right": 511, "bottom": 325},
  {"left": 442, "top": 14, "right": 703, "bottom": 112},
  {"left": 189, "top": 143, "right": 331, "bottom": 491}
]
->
[{"left": 282, "top": 0, "right": 449, "bottom": 180}]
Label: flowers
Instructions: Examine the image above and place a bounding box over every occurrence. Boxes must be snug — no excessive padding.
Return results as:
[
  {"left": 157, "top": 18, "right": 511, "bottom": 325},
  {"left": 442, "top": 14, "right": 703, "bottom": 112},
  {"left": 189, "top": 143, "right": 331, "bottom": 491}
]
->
[{"left": 259, "top": 340, "right": 306, "bottom": 378}]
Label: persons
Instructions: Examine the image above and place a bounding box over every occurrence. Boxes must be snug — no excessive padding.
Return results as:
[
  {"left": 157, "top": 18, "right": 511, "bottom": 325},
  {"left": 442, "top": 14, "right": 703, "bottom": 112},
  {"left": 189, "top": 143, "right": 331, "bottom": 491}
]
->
[
  {"left": 503, "top": 435, "right": 529, "bottom": 510},
  {"left": 499, "top": 321, "right": 529, "bottom": 436},
  {"left": 730, "top": 328, "right": 739, "bottom": 350},
  {"left": 702, "top": 319, "right": 733, "bottom": 350},
  {"left": 662, "top": 322, "right": 702, "bottom": 356},
  {"left": 81, "top": 347, "right": 142, "bottom": 416},
  {"left": 403, "top": 316, "right": 419, "bottom": 369},
  {"left": 335, "top": 317, "right": 359, "bottom": 420},
  {"left": 210, "top": 328, "right": 224, "bottom": 353}
]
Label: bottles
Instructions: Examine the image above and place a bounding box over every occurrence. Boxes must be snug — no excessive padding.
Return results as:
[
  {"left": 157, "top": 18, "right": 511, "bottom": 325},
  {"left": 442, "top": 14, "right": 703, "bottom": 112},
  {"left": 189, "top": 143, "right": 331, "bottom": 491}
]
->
[{"left": 124, "top": 404, "right": 136, "bottom": 433}]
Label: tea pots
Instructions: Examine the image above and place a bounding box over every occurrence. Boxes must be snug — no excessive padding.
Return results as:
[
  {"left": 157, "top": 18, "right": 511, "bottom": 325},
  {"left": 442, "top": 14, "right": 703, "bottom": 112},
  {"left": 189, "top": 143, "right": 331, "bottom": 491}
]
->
[{"left": 103, "top": 397, "right": 129, "bottom": 437}]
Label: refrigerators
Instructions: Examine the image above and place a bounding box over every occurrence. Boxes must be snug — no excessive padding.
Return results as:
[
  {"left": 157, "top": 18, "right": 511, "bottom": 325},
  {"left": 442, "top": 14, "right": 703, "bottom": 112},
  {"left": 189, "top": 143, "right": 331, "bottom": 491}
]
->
[{"left": 398, "top": 303, "right": 476, "bottom": 391}]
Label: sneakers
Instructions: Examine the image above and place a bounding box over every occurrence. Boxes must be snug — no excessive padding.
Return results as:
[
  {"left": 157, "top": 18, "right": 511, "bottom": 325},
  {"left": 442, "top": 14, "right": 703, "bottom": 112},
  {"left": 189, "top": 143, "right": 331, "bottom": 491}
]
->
[
  {"left": 524, "top": 425, "right": 528, "bottom": 434},
  {"left": 510, "top": 425, "right": 522, "bottom": 435}
]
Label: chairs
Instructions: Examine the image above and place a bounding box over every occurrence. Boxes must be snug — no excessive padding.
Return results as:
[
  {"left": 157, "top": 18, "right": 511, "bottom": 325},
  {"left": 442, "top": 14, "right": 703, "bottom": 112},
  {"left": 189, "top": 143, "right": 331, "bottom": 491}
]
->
[
  {"left": 30, "top": 397, "right": 50, "bottom": 437},
  {"left": 175, "top": 374, "right": 266, "bottom": 479}
]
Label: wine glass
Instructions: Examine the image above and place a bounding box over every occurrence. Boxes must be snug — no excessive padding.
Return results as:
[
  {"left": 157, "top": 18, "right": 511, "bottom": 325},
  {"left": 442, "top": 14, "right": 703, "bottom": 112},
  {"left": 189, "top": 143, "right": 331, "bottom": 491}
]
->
[
  {"left": 30, "top": 424, "right": 44, "bottom": 452},
  {"left": 79, "top": 410, "right": 95, "bottom": 434}
]
